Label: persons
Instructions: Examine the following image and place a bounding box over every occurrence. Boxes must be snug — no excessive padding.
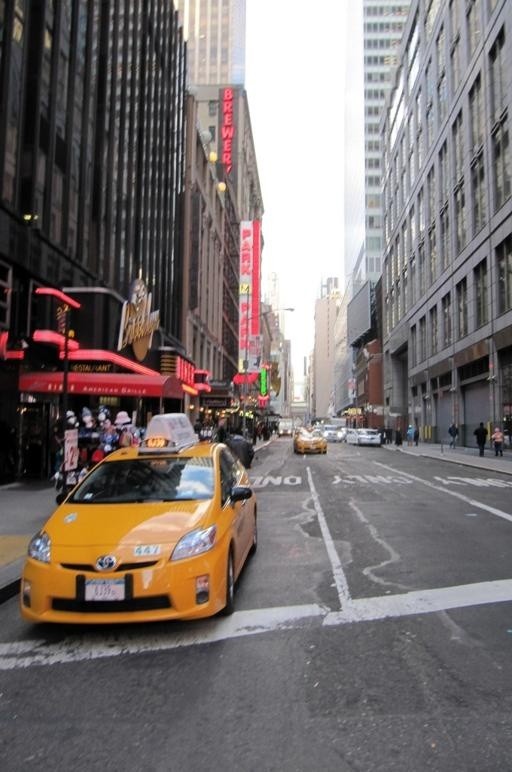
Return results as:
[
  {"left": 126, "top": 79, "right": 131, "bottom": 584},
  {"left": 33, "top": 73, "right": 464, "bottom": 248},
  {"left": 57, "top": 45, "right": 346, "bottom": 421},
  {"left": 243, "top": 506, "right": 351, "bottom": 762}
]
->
[
  {"left": 372, "top": 420, "right": 508, "bottom": 458},
  {"left": 174, "top": 417, "right": 279, "bottom": 495},
  {"left": 51, "top": 406, "right": 139, "bottom": 487}
]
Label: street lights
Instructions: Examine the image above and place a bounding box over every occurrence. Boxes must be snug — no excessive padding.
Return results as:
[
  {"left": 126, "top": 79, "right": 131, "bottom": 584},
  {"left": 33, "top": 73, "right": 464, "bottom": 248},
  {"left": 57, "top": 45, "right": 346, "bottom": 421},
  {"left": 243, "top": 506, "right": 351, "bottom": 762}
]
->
[{"left": 243, "top": 284, "right": 294, "bottom": 439}]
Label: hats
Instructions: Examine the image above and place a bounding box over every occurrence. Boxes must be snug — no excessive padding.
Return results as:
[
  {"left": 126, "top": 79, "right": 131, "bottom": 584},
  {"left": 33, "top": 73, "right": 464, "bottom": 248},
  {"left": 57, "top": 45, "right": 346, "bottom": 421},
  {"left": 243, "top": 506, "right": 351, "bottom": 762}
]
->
[
  {"left": 64, "top": 409, "right": 77, "bottom": 419},
  {"left": 114, "top": 410, "right": 133, "bottom": 424},
  {"left": 99, "top": 407, "right": 111, "bottom": 420},
  {"left": 81, "top": 405, "right": 93, "bottom": 418}
]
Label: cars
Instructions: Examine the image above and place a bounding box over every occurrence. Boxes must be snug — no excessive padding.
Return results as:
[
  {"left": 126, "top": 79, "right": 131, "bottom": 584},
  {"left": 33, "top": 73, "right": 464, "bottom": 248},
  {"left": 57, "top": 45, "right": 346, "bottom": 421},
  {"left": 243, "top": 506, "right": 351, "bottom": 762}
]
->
[
  {"left": 195, "top": 426, "right": 254, "bottom": 469},
  {"left": 275, "top": 417, "right": 381, "bottom": 455},
  {"left": 19, "top": 412, "right": 258, "bottom": 624}
]
